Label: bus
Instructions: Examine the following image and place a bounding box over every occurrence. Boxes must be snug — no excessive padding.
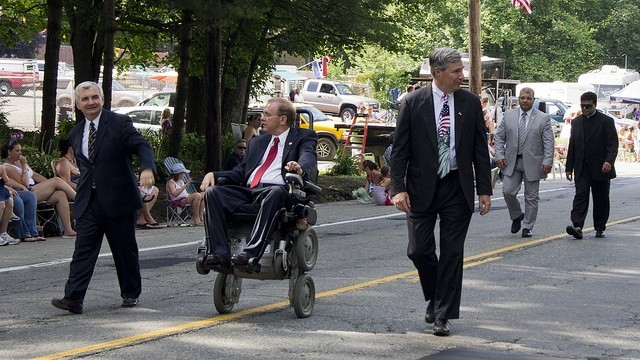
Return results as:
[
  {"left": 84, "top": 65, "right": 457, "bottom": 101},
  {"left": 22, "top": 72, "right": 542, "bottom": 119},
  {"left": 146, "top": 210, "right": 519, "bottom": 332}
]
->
[
  {"left": 419, "top": 53, "right": 505, "bottom": 90},
  {"left": 515, "top": 82, "right": 599, "bottom": 114},
  {"left": 579, "top": 65, "right": 639, "bottom": 99}
]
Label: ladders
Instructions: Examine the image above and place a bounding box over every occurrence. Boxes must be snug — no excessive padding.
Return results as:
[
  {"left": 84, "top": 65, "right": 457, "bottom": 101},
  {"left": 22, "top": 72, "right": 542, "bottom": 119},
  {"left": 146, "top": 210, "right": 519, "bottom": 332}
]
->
[{"left": 341, "top": 111, "right": 369, "bottom": 177}]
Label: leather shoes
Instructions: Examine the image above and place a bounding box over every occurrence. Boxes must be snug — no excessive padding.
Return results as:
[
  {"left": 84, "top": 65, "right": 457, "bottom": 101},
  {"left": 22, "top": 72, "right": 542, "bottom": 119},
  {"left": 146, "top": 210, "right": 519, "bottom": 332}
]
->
[
  {"left": 566, "top": 225, "right": 583, "bottom": 240},
  {"left": 511, "top": 213, "right": 524, "bottom": 234},
  {"left": 595, "top": 232, "right": 605, "bottom": 238},
  {"left": 426, "top": 299, "right": 435, "bottom": 323},
  {"left": 231, "top": 252, "right": 254, "bottom": 269},
  {"left": 121, "top": 297, "right": 140, "bottom": 307},
  {"left": 522, "top": 229, "right": 532, "bottom": 237},
  {"left": 433, "top": 319, "right": 451, "bottom": 336},
  {"left": 51, "top": 297, "right": 83, "bottom": 314},
  {"left": 206, "top": 253, "right": 233, "bottom": 272}
]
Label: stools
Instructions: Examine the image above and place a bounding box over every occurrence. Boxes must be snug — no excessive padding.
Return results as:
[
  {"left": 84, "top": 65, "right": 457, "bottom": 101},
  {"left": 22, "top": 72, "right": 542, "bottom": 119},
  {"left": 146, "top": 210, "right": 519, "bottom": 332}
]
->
[
  {"left": 618, "top": 148, "right": 632, "bottom": 163},
  {"left": 544, "top": 160, "right": 562, "bottom": 180}
]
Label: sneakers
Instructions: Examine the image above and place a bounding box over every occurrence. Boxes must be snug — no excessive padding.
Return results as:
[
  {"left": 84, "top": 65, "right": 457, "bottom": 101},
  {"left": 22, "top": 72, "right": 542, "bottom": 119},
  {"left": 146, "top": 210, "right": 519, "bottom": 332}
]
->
[
  {"left": 0, "top": 235, "right": 9, "bottom": 246},
  {"left": 1, "top": 232, "right": 21, "bottom": 245}
]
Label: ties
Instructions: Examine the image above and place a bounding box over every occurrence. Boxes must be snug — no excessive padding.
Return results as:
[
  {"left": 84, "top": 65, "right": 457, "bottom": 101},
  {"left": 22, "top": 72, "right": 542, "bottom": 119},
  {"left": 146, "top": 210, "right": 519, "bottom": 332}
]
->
[
  {"left": 436, "top": 92, "right": 451, "bottom": 178},
  {"left": 250, "top": 136, "right": 280, "bottom": 189},
  {"left": 518, "top": 112, "right": 527, "bottom": 155},
  {"left": 88, "top": 121, "right": 97, "bottom": 189}
]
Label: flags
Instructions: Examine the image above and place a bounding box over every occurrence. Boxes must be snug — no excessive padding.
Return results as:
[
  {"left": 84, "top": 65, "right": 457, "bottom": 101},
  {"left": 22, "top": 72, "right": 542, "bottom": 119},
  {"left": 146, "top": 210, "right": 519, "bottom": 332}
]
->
[{"left": 311, "top": 58, "right": 328, "bottom": 79}]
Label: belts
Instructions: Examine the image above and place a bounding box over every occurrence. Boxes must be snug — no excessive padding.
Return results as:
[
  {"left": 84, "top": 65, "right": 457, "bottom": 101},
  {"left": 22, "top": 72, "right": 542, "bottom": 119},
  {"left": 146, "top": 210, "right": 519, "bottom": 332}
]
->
[{"left": 250, "top": 181, "right": 275, "bottom": 190}]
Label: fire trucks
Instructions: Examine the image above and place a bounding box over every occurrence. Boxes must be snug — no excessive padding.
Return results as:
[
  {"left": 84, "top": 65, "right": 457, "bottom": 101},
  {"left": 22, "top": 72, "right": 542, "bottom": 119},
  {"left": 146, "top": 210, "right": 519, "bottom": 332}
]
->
[{"left": 0, "top": 60, "right": 39, "bottom": 98}]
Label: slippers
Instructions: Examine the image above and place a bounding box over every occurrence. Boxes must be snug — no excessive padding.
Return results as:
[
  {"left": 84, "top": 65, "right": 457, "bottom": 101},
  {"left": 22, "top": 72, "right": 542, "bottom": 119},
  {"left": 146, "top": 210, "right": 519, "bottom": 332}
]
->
[
  {"left": 149, "top": 223, "right": 164, "bottom": 229},
  {"left": 21, "top": 235, "right": 37, "bottom": 242},
  {"left": 34, "top": 235, "right": 46, "bottom": 241},
  {"left": 136, "top": 221, "right": 153, "bottom": 229}
]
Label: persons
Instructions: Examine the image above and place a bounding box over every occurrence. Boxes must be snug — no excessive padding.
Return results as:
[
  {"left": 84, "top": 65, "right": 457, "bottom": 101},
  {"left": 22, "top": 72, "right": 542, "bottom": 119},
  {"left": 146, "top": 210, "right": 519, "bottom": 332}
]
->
[
  {"left": 13, "top": 191, "right": 46, "bottom": 243},
  {"left": 627, "top": 127, "right": 637, "bottom": 162},
  {"left": 373, "top": 167, "right": 394, "bottom": 206},
  {"left": 135, "top": 186, "right": 163, "bottom": 229},
  {"left": 357, "top": 104, "right": 366, "bottom": 120},
  {"left": 494, "top": 88, "right": 554, "bottom": 239},
  {"left": 166, "top": 162, "right": 205, "bottom": 226},
  {"left": 620, "top": 128, "right": 635, "bottom": 157},
  {"left": 388, "top": 47, "right": 493, "bottom": 336},
  {"left": 292, "top": 90, "right": 304, "bottom": 103},
  {"left": 160, "top": 107, "right": 172, "bottom": 132},
  {"left": 565, "top": 90, "right": 619, "bottom": 240},
  {"left": 637, "top": 120, "right": 640, "bottom": 165},
  {"left": 368, "top": 105, "right": 383, "bottom": 121},
  {"left": 481, "top": 96, "right": 493, "bottom": 108},
  {"left": 1, "top": 165, "right": 21, "bottom": 247},
  {"left": 363, "top": 161, "right": 381, "bottom": 192},
  {"left": 287, "top": 89, "right": 292, "bottom": 100},
  {"left": 199, "top": 98, "right": 318, "bottom": 277},
  {"left": 224, "top": 140, "right": 247, "bottom": 170},
  {"left": 246, "top": 114, "right": 263, "bottom": 143},
  {"left": 632, "top": 108, "right": 640, "bottom": 120},
  {"left": 0, "top": 140, "right": 79, "bottom": 238},
  {"left": 51, "top": 82, "right": 156, "bottom": 315},
  {"left": 57, "top": 139, "right": 82, "bottom": 190},
  {"left": 481, "top": 108, "right": 497, "bottom": 143}
]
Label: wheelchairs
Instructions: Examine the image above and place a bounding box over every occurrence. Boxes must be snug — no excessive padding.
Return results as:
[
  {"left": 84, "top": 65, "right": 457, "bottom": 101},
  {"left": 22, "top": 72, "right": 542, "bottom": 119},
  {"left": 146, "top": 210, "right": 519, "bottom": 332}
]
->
[{"left": 196, "top": 108, "right": 322, "bottom": 318}]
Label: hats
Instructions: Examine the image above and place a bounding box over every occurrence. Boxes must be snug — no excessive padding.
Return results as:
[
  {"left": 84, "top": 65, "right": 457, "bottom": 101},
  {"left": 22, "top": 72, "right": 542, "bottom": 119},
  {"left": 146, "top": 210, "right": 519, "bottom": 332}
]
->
[{"left": 171, "top": 163, "right": 190, "bottom": 174}]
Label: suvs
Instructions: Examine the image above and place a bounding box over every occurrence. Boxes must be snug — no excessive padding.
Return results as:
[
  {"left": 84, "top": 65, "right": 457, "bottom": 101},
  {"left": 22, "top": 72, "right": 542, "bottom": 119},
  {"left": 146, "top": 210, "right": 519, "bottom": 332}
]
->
[
  {"left": 133, "top": 91, "right": 176, "bottom": 108},
  {"left": 56, "top": 77, "right": 146, "bottom": 109},
  {"left": 295, "top": 79, "right": 380, "bottom": 123}
]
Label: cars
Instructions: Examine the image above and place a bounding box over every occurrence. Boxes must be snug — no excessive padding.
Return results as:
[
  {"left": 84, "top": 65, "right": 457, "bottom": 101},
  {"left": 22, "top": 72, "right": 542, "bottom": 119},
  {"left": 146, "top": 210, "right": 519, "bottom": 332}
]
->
[
  {"left": 38, "top": 61, "right": 75, "bottom": 82},
  {"left": 247, "top": 103, "right": 349, "bottom": 162},
  {"left": 110, "top": 105, "right": 175, "bottom": 134}
]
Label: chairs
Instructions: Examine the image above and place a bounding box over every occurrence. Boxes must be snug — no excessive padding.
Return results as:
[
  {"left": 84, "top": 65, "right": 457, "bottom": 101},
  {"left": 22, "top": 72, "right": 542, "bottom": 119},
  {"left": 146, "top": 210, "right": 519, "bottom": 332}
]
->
[
  {"left": 166, "top": 180, "right": 193, "bottom": 228},
  {"left": 164, "top": 157, "right": 198, "bottom": 194},
  {"left": 36, "top": 200, "right": 64, "bottom": 239},
  {"left": 51, "top": 157, "right": 81, "bottom": 233}
]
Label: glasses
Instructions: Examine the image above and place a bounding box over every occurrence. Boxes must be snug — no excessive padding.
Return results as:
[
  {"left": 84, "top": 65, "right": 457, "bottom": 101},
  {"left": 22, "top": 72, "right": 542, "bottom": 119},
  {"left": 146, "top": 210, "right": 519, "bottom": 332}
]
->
[
  {"left": 260, "top": 111, "right": 281, "bottom": 120},
  {"left": 580, "top": 104, "right": 594, "bottom": 109},
  {"left": 237, "top": 146, "right": 247, "bottom": 150}
]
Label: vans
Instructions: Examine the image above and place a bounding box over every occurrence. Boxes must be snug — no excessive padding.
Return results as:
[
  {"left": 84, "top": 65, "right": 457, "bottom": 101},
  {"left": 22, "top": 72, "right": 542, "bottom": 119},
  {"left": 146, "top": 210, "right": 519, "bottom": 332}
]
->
[{"left": 496, "top": 98, "right": 569, "bottom": 124}]
